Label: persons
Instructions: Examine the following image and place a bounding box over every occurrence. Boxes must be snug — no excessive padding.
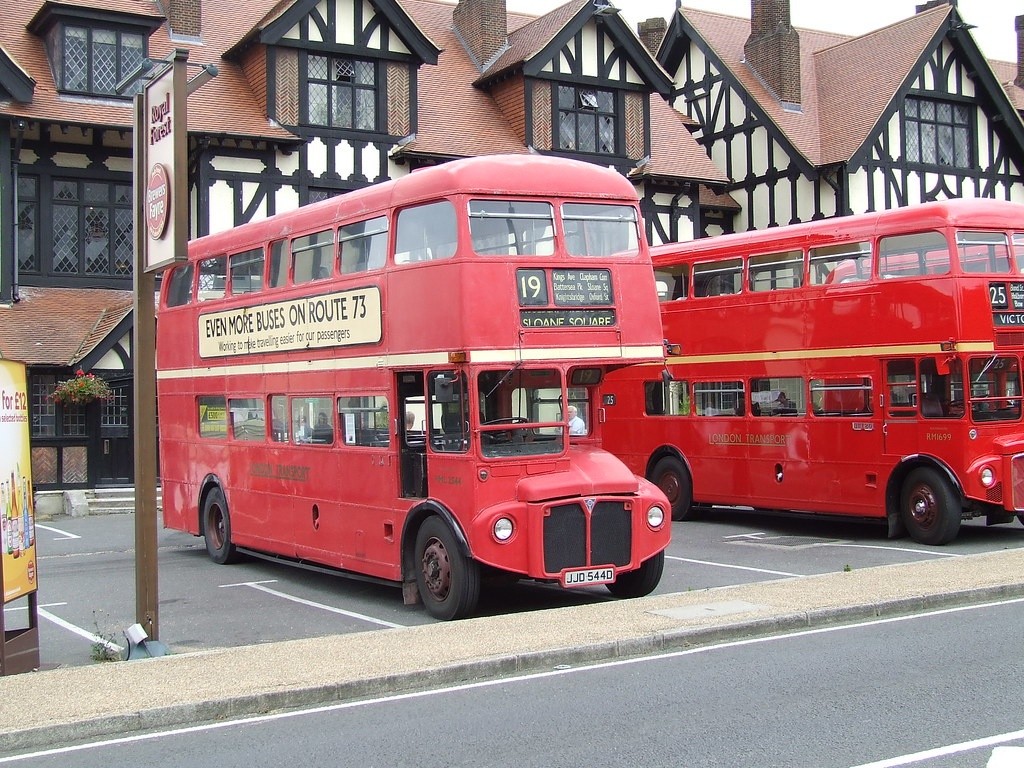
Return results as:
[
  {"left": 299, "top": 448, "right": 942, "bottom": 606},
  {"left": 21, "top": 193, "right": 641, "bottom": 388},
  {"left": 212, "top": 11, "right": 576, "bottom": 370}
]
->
[
  {"left": 560, "top": 405, "right": 587, "bottom": 435},
  {"left": 312, "top": 412, "right": 333, "bottom": 444},
  {"left": 771, "top": 392, "right": 796, "bottom": 416},
  {"left": 405, "top": 411, "right": 414, "bottom": 429}
]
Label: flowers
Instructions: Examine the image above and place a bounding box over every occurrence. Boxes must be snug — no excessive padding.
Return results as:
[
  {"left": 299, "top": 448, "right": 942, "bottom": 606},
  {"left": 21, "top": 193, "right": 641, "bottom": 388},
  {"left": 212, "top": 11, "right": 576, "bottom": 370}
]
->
[{"left": 46, "top": 368, "right": 114, "bottom": 405}]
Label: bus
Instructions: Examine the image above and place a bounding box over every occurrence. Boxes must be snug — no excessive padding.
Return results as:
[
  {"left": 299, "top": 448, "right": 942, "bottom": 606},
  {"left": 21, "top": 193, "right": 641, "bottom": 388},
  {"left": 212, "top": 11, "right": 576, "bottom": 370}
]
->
[
  {"left": 586, "top": 197, "right": 1024, "bottom": 547},
  {"left": 153, "top": 157, "right": 683, "bottom": 622}
]
